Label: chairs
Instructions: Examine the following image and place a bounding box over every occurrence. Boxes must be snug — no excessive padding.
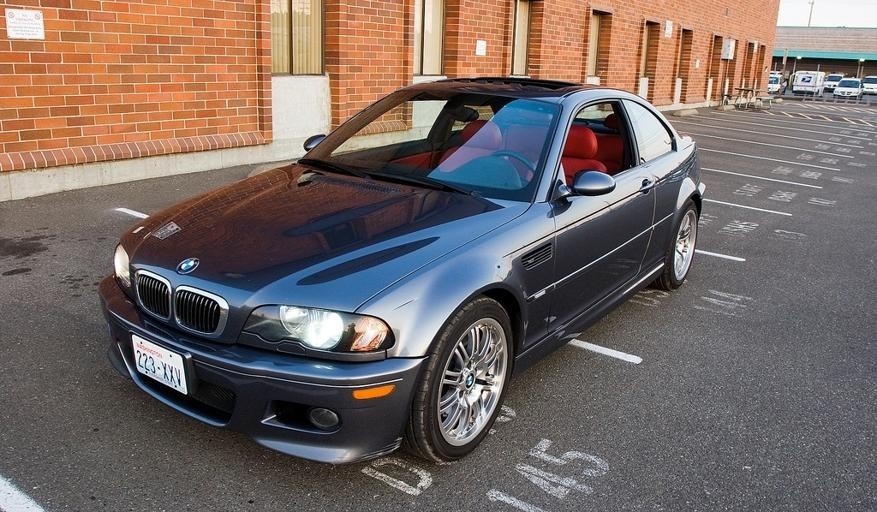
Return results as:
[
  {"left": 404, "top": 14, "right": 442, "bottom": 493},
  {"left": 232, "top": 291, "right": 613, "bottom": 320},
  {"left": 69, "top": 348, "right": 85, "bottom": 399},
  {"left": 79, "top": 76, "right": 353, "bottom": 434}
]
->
[{"left": 437, "top": 114, "right": 625, "bottom": 187}]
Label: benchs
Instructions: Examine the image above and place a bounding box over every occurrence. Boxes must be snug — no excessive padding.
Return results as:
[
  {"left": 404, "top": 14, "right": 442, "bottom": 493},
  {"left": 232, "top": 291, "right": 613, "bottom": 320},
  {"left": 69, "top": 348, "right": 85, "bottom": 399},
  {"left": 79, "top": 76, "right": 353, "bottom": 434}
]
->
[{"left": 721, "top": 95, "right": 774, "bottom": 110}]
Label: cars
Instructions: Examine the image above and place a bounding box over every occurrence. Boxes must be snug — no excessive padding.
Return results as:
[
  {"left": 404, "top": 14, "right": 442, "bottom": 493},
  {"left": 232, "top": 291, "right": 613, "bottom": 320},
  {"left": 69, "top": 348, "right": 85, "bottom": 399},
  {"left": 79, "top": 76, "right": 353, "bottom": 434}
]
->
[{"left": 90, "top": 69, "right": 706, "bottom": 474}]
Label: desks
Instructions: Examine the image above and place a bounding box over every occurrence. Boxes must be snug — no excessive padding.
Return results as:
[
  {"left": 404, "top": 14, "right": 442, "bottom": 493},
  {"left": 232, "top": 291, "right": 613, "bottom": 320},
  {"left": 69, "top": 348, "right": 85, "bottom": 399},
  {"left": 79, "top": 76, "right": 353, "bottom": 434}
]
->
[{"left": 733, "top": 88, "right": 765, "bottom": 110}]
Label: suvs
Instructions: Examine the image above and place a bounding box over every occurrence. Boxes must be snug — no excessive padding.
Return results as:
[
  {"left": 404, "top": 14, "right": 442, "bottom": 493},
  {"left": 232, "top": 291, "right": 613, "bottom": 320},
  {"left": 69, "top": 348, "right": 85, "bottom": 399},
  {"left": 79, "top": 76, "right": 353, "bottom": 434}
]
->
[{"left": 767, "top": 67, "right": 877, "bottom": 102}]
